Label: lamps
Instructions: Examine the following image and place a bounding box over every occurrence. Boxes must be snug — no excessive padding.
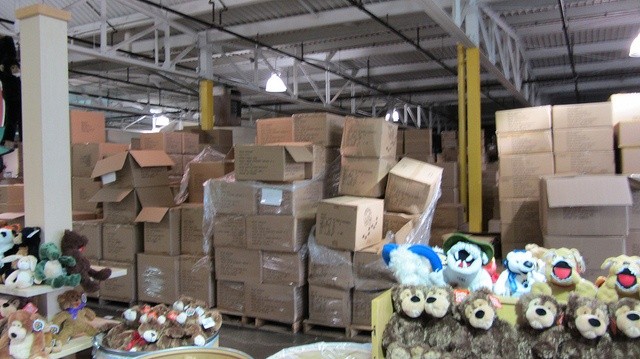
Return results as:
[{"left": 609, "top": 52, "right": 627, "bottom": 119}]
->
[
  {"left": 260, "top": 53, "right": 286, "bottom": 94},
  {"left": 385, "top": 100, "right": 400, "bottom": 124},
  {"left": 628, "top": 28, "right": 640, "bottom": 59}
]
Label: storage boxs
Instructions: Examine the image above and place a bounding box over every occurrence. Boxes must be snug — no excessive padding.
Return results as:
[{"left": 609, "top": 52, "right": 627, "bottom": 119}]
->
[
  {"left": 0, "top": 170, "right": 23, "bottom": 228},
  {"left": 490, "top": 90, "right": 640, "bottom": 290},
  {"left": 207, "top": 111, "right": 341, "bottom": 335},
  {"left": 392, "top": 126, "right": 489, "bottom": 262},
  {"left": 67, "top": 107, "right": 141, "bottom": 308},
  {"left": 304, "top": 115, "right": 444, "bottom": 339},
  {"left": 130, "top": 131, "right": 252, "bottom": 311}
]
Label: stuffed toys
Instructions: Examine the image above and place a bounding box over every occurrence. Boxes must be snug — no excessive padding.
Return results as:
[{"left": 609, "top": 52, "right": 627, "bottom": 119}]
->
[
  {"left": 442, "top": 234, "right": 494, "bottom": 303},
  {"left": 30, "top": 313, "right": 53, "bottom": 355},
  {"left": 0, "top": 227, "right": 42, "bottom": 285},
  {"left": 0, "top": 222, "right": 21, "bottom": 269},
  {"left": 424, "top": 286, "right": 460, "bottom": 359},
  {"left": 455, "top": 286, "right": 518, "bottom": 359},
  {"left": 61, "top": 229, "right": 113, "bottom": 293},
  {"left": 0, "top": 34, "right": 23, "bottom": 182},
  {"left": 493, "top": 249, "right": 547, "bottom": 297},
  {"left": 526, "top": 243, "right": 606, "bottom": 305},
  {"left": 35, "top": 243, "right": 81, "bottom": 288},
  {"left": 98, "top": 295, "right": 222, "bottom": 352},
  {"left": 609, "top": 298, "right": 640, "bottom": 359},
  {"left": 1, "top": 255, "right": 38, "bottom": 290},
  {"left": 512, "top": 293, "right": 565, "bottom": 359},
  {"left": 381, "top": 244, "right": 446, "bottom": 290},
  {"left": 50, "top": 290, "right": 100, "bottom": 353},
  {"left": 595, "top": 255, "right": 640, "bottom": 304},
  {"left": 0, "top": 296, "right": 39, "bottom": 339},
  {"left": 381, "top": 283, "right": 425, "bottom": 359},
  {"left": 0, "top": 295, "right": 14, "bottom": 307},
  {"left": 559, "top": 296, "right": 614, "bottom": 359},
  {"left": 0, "top": 310, "right": 50, "bottom": 359},
  {"left": 482, "top": 258, "right": 499, "bottom": 284}
]
